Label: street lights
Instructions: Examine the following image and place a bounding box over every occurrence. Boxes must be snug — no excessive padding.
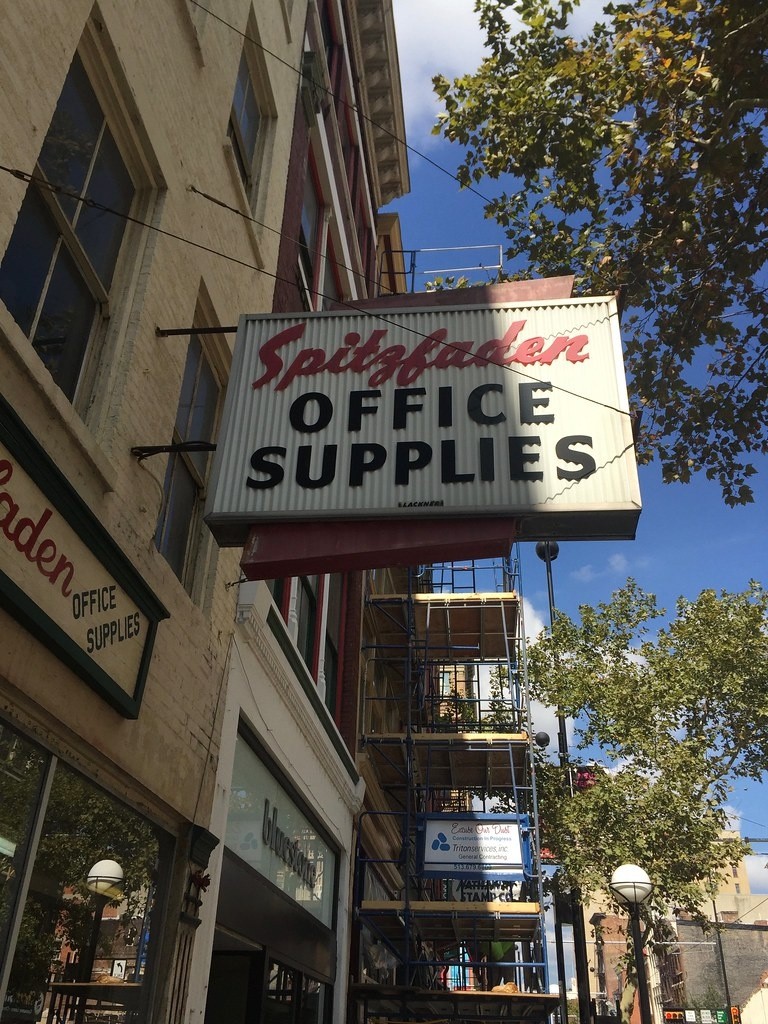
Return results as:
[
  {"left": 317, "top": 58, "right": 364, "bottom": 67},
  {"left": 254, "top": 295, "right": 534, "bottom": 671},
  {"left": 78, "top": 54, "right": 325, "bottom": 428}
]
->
[
  {"left": 71, "top": 859, "right": 127, "bottom": 1024},
  {"left": 536, "top": 539, "right": 598, "bottom": 1024},
  {"left": 609, "top": 863, "right": 657, "bottom": 1023}
]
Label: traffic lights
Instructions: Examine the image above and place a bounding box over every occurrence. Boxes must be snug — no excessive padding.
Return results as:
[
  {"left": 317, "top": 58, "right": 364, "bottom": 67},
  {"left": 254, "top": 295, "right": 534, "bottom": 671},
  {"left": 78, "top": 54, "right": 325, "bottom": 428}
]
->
[
  {"left": 731, "top": 1006, "right": 741, "bottom": 1024},
  {"left": 662, "top": 1009, "right": 684, "bottom": 1020}
]
